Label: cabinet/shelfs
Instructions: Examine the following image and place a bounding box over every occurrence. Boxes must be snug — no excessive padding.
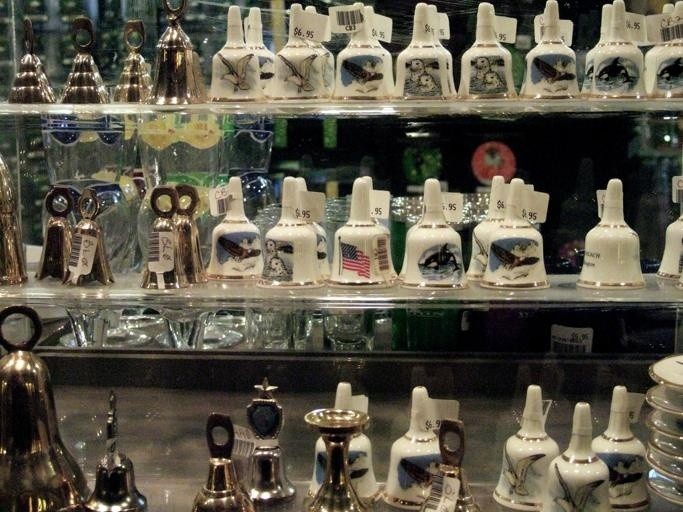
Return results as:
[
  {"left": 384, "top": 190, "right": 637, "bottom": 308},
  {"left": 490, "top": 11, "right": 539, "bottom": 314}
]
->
[{"left": 1, "top": 97, "right": 683, "bottom": 368}]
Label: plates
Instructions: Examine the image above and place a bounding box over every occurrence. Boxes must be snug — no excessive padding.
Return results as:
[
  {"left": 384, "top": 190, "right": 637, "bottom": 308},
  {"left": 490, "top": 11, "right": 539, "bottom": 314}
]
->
[{"left": 644, "top": 353, "right": 683, "bottom": 512}]
